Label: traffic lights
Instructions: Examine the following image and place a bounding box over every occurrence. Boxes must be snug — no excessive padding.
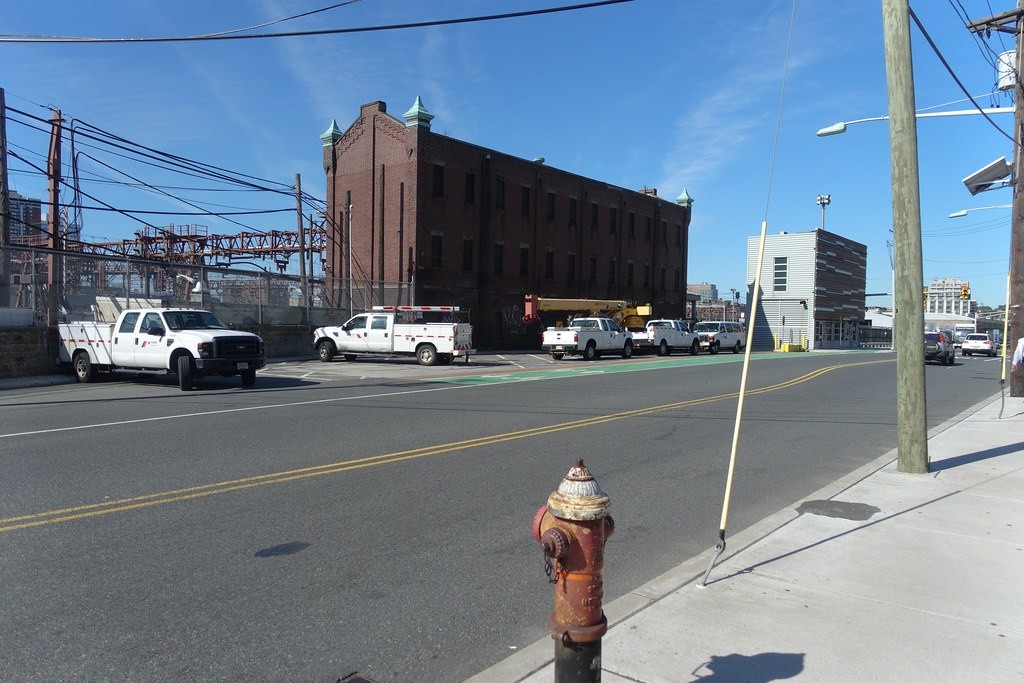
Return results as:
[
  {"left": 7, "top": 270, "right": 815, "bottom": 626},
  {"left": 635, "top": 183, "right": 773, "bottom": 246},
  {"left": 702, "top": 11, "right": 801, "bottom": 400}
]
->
[{"left": 962, "top": 285, "right": 969, "bottom": 299}]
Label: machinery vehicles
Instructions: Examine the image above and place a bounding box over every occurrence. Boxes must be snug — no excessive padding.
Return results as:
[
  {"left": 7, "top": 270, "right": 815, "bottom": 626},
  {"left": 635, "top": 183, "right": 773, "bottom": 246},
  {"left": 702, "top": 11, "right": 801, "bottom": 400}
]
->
[{"left": 522, "top": 293, "right": 653, "bottom": 332}]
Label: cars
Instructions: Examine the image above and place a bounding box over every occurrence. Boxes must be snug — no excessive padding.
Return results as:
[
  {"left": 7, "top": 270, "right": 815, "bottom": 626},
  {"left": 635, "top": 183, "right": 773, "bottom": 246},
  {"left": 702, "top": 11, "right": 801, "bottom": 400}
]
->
[
  {"left": 994, "top": 337, "right": 1012, "bottom": 349},
  {"left": 961, "top": 333, "right": 998, "bottom": 358}
]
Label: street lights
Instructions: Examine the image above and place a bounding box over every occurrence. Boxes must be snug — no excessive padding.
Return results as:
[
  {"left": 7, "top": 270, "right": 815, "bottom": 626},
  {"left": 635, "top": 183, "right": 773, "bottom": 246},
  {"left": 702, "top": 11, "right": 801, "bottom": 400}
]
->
[
  {"left": 349, "top": 204, "right": 354, "bottom": 319},
  {"left": 730, "top": 289, "right": 737, "bottom": 322},
  {"left": 815, "top": 102, "right": 1024, "bottom": 398},
  {"left": 816, "top": 194, "right": 831, "bottom": 231}
]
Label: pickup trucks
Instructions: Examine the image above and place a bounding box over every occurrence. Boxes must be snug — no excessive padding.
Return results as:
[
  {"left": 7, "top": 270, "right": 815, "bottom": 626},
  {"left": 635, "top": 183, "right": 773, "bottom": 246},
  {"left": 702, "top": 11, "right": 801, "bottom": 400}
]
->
[
  {"left": 540, "top": 318, "right": 633, "bottom": 361},
  {"left": 624, "top": 319, "right": 700, "bottom": 357},
  {"left": 314, "top": 312, "right": 477, "bottom": 366},
  {"left": 57, "top": 308, "right": 268, "bottom": 391}
]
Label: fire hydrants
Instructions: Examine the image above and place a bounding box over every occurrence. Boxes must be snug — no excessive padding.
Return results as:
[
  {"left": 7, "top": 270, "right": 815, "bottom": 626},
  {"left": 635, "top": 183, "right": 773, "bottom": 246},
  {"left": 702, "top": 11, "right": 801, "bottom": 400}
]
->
[{"left": 531, "top": 457, "right": 615, "bottom": 683}]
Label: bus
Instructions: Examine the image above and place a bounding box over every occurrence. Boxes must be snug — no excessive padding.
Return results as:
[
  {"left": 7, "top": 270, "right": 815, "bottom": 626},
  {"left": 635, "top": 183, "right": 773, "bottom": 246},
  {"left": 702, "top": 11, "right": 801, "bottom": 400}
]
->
[{"left": 952, "top": 324, "right": 976, "bottom": 349}]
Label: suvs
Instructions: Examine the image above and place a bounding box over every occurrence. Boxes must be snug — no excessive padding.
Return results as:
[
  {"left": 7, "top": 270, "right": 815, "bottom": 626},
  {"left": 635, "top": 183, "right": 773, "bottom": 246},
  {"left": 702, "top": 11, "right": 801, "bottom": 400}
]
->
[
  {"left": 693, "top": 322, "right": 746, "bottom": 355},
  {"left": 924, "top": 330, "right": 955, "bottom": 367}
]
